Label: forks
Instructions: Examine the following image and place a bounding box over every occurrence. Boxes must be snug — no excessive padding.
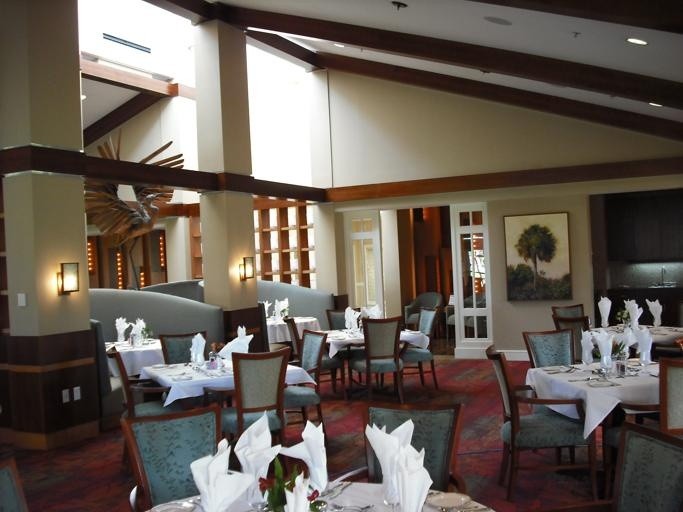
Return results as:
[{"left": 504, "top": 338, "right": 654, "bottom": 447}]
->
[{"left": 547, "top": 367, "right": 575, "bottom": 374}]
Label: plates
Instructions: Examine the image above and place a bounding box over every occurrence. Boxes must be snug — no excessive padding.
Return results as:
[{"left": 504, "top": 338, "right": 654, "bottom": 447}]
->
[
  {"left": 542, "top": 366, "right": 561, "bottom": 371},
  {"left": 150, "top": 363, "right": 192, "bottom": 381},
  {"left": 426, "top": 491, "right": 470, "bottom": 507},
  {"left": 149, "top": 501, "right": 195, "bottom": 511},
  {"left": 315, "top": 329, "right": 363, "bottom": 340},
  {"left": 587, "top": 380, "right": 614, "bottom": 387}
]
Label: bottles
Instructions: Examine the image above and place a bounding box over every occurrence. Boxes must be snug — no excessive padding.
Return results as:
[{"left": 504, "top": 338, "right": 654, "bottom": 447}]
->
[
  {"left": 129, "top": 333, "right": 137, "bottom": 347},
  {"left": 616, "top": 354, "right": 626, "bottom": 377}
]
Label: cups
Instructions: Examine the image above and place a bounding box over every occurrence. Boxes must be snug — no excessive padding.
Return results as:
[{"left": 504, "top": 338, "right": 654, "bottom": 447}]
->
[{"left": 246, "top": 485, "right": 269, "bottom": 512}]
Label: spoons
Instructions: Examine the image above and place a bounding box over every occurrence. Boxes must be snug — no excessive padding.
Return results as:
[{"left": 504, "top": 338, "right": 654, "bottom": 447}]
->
[
  {"left": 568, "top": 376, "right": 592, "bottom": 382},
  {"left": 328, "top": 501, "right": 373, "bottom": 511}
]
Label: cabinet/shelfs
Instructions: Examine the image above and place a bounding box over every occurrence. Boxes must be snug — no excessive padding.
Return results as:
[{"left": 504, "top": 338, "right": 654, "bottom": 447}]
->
[{"left": 601, "top": 186, "right": 683, "bottom": 264}]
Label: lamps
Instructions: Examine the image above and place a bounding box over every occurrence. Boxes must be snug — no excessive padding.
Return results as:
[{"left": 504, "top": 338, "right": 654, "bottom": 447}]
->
[
  {"left": 237, "top": 256, "right": 254, "bottom": 282},
  {"left": 55, "top": 260, "right": 81, "bottom": 298}
]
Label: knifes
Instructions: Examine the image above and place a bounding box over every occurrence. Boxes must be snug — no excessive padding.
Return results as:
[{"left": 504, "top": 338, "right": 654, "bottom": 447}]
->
[{"left": 320, "top": 481, "right": 351, "bottom": 500}]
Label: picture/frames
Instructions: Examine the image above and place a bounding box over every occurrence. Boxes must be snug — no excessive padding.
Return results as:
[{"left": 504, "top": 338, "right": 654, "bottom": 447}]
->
[{"left": 501, "top": 209, "right": 573, "bottom": 303}]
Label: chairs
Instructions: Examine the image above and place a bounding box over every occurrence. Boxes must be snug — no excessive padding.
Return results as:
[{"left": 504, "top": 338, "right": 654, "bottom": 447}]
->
[{"left": 0, "top": 453, "right": 30, "bottom": 511}]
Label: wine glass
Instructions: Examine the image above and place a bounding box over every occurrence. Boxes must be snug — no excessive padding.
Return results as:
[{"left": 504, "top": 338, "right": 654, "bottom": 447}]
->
[
  {"left": 639, "top": 351, "right": 650, "bottom": 373},
  {"left": 599, "top": 355, "right": 612, "bottom": 381},
  {"left": 381, "top": 479, "right": 400, "bottom": 512}
]
